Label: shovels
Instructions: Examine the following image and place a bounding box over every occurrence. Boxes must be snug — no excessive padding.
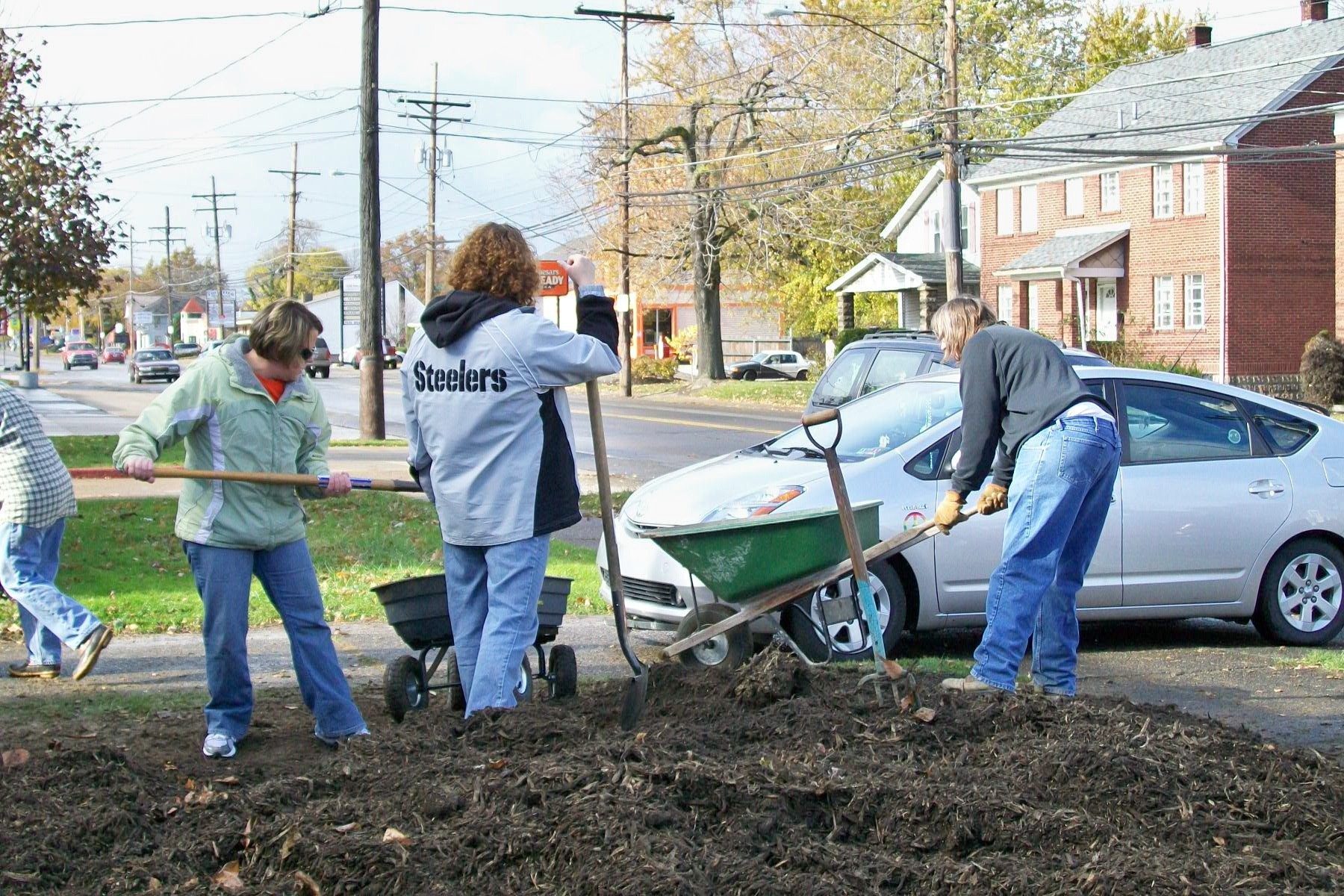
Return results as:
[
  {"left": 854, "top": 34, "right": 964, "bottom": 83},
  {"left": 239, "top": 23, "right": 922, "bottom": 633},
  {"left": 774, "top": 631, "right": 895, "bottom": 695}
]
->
[{"left": 585, "top": 380, "right": 648, "bottom": 730}]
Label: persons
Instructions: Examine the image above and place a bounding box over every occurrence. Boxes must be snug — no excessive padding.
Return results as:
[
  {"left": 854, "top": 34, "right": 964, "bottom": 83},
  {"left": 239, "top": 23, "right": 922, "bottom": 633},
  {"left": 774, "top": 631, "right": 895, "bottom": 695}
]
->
[
  {"left": 403, "top": 222, "right": 622, "bottom": 716},
  {"left": 0, "top": 379, "right": 115, "bottom": 681},
  {"left": 112, "top": 298, "right": 371, "bottom": 758},
  {"left": 930, "top": 295, "right": 1122, "bottom": 700}
]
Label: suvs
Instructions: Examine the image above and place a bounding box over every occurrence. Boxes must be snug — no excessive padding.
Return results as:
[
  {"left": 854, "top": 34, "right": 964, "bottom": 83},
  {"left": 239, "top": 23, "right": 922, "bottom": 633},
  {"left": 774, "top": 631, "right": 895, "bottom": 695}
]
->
[
  {"left": 304, "top": 335, "right": 333, "bottom": 379},
  {"left": 802, "top": 328, "right": 1117, "bottom": 424},
  {"left": 61, "top": 341, "right": 100, "bottom": 371}
]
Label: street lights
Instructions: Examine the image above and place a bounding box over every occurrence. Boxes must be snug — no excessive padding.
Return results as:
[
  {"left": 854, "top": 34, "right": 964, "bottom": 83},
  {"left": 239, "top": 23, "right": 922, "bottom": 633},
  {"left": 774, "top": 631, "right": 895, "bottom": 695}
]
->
[
  {"left": 330, "top": 169, "right": 437, "bottom": 306},
  {"left": 764, "top": 8, "right": 964, "bottom": 304}
]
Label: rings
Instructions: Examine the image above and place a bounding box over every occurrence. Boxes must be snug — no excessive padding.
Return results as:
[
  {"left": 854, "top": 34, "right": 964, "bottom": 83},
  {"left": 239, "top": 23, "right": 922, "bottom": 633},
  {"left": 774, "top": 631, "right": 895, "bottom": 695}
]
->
[{"left": 334, "top": 490, "right": 339, "bottom": 493}]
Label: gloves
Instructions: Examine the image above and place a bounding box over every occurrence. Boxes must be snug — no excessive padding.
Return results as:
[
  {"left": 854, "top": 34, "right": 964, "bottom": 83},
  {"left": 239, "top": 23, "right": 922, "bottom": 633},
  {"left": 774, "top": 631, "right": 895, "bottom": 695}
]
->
[
  {"left": 934, "top": 492, "right": 968, "bottom": 534},
  {"left": 977, "top": 483, "right": 1009, "bottom": 514}
]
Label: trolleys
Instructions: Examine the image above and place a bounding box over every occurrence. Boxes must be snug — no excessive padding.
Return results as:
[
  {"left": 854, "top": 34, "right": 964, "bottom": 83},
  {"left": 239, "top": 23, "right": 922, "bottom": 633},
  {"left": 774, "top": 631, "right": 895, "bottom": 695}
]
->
[{"left": 370, "top": 572, "right": 580, "bottom": 724}]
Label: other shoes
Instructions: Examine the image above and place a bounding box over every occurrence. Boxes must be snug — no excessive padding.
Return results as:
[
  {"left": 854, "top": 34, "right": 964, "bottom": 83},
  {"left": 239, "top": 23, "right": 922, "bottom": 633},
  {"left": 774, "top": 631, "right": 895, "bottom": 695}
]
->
[
  {"left": 8, "top": 663, "right": 61, "bottom": 679},
  {"left": 72, "top": 624, "right": 113, "bottom": 681}
]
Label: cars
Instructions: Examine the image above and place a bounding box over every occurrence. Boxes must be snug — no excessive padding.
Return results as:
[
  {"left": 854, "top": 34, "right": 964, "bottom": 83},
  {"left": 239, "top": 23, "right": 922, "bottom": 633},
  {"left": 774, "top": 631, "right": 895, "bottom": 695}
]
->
[
  {"left": 127, "top": 347, "right": 181, "bottom": 385},
  {"left": 338, "top": 339, "right": 406, "bottom": 366},
  {"left": 143, "top": 342, "right": 201, "bottom": 358},
  {"left": 196, "top": 340, "right": 224, "bottom": 356},
  {"left": 725, "top": 350, "right": 818, "bottom": 383},
  {"left": 101, "top": 347, "right": 126, "bottom": 365},
  {"left": 597, "top": 367, "right": 1344, "bottom": 661}
]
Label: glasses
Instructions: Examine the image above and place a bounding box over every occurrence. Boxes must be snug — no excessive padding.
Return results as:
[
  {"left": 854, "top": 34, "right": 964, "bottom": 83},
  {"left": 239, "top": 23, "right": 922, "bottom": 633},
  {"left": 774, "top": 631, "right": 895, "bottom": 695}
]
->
[{"left": 299, "top": 344, "right": 319, "bottom": 362}]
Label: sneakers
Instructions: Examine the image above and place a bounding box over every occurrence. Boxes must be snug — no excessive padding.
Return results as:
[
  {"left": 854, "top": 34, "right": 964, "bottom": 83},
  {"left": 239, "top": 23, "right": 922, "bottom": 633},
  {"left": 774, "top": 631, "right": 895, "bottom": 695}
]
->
[
  {"left": 942, "top": 676, "right": 993, "bottom": 690},
  {"left": 203, "top": 734, "right": 237, "bottom": 758},
  {"left": 314, "top": 728, "right": 371, "bottom": 745}
]
